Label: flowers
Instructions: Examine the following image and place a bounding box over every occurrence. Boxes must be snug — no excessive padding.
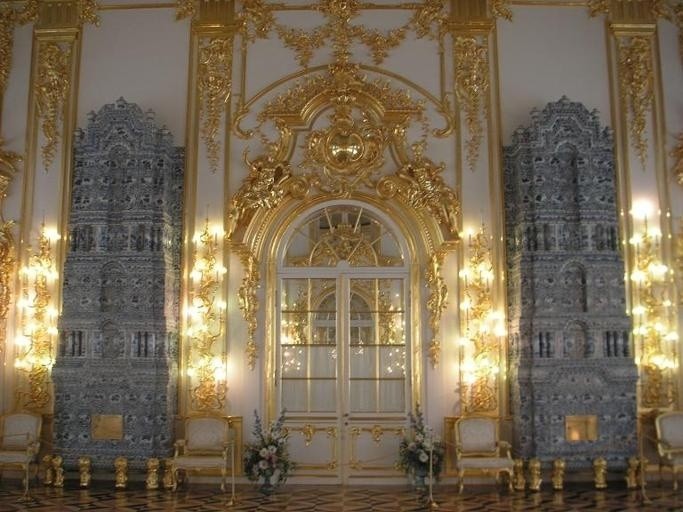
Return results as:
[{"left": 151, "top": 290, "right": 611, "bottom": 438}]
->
[
  {"left": 397, "top": 404, "right": 448, "bottom": 484},
  {"left": 241, "top": 408, "right": 296, "bottom": 491}
]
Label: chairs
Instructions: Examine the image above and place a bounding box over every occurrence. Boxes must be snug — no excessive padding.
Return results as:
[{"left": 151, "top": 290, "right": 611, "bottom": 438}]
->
[
  {"left": 0, "top": 410, "right": 45, "bottom": 488},
  {"left": 652, "top": 411, "right": 683, "bottom": 496},
  {"left": 453, "top": 415, "right": 517, "bottom": 496},
  {"left": 169, "top": 413, "right": 236, "bottom": 493}
]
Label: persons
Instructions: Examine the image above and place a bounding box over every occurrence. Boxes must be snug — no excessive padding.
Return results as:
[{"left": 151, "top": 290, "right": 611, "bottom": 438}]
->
[
  {"left": 224, "top": 143, "right": 288, "bottom": 240},
  {"left": 395, "top": 143, "right": 460, "bottom": 240}
]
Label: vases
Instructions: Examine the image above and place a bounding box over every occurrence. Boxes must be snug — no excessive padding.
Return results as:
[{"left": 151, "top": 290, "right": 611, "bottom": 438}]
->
[
  {"left": 413, "top": 469, "right": 428, "bottom": 491},
  {"left": 259, "top": 469, "right": 274, "bottom": 490}
]
